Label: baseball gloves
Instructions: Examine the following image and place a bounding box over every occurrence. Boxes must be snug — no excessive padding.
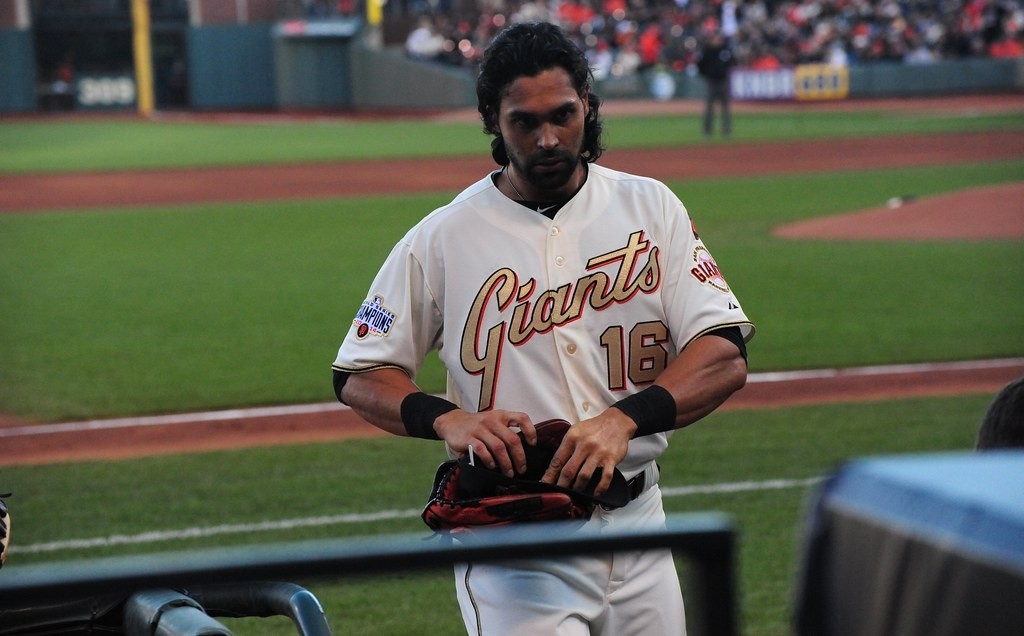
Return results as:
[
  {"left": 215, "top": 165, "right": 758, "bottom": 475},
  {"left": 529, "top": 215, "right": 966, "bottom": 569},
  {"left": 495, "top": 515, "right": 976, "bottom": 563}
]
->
[{"left": 421, "top": 416, "right": 632, "bottom": 532}]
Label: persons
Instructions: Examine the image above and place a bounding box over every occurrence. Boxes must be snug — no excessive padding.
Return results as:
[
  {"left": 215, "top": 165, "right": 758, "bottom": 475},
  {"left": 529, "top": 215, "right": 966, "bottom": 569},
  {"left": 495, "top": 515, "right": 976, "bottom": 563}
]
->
[
  {"left": 698, "top": 28, "right": 735, "bottom": 136},
  {"left": 404, "top": 0, "right": 1024, "bottom": 97},
  {"left": 332, "top": 20, "right": 756, "bottom": 636}
]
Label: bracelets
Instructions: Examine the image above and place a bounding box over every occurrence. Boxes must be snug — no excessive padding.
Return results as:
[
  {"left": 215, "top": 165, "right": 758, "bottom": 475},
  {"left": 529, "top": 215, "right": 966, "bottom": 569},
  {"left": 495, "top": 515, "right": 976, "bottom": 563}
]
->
[
  {"left": 400, "top": 392, "right": 462, "bottom": 441},
  {"left": 610, "top": 384, "right": 677, "bottom": 440}
]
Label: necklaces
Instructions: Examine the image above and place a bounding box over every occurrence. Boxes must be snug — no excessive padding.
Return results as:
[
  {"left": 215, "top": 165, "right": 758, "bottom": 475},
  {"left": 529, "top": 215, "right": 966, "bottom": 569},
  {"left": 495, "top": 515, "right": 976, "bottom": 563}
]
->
[{"left": 505, "top": 164, "right": 525, "bottom": 200}]
